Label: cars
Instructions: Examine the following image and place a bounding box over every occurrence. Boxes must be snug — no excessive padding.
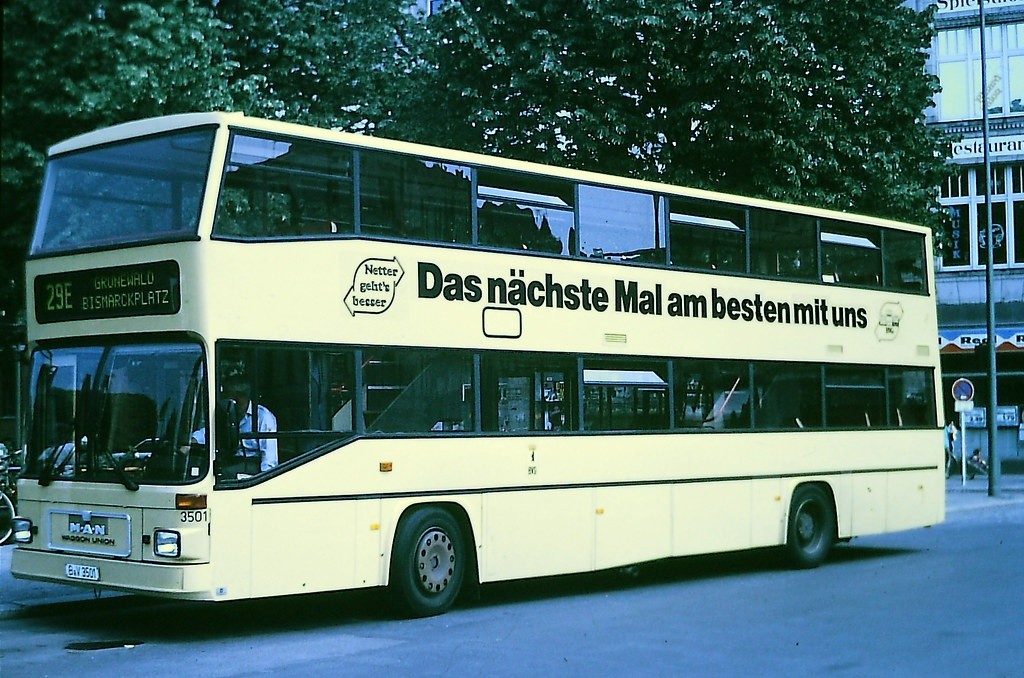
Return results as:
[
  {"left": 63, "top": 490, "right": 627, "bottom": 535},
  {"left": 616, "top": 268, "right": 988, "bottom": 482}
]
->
[{"left": 111, "top": 436, "right": 160, "bottom": 459}]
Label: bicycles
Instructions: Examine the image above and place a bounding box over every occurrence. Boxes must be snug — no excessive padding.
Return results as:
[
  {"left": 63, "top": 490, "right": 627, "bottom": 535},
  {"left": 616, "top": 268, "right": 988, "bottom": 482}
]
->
[{"left": 0, "top": 482, "right": 17, "bottom": 545}]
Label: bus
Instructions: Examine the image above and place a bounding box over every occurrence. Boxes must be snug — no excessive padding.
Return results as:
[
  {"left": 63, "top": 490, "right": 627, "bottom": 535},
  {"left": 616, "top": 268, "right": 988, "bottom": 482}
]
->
[{"left": 8, "top": 109, "right": 950, "bottom": 618}]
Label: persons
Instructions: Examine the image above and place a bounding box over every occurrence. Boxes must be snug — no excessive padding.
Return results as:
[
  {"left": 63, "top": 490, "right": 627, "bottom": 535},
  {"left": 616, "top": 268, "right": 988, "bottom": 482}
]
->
[{"left": 187, "top": 374, "right": 278, "bottom": 474}]
